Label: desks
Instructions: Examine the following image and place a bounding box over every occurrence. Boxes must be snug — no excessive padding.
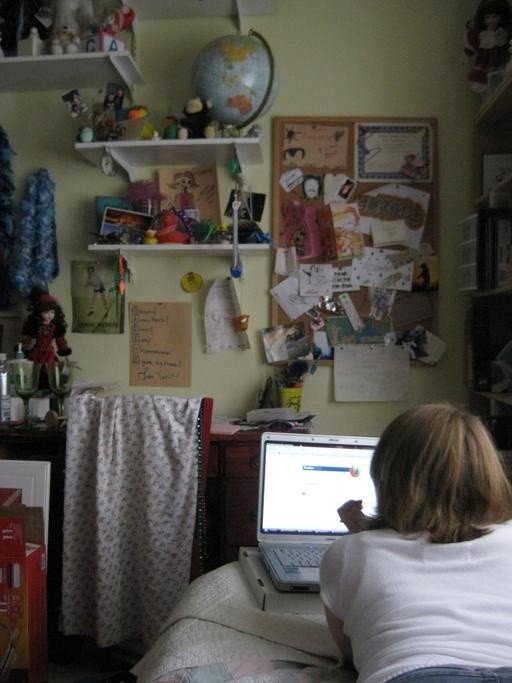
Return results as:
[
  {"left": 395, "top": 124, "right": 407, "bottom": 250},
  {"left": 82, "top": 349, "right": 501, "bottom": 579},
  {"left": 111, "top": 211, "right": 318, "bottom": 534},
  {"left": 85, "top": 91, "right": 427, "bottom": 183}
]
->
[{"left": 0, "top": 416, "right": 305, "bottom": 569}]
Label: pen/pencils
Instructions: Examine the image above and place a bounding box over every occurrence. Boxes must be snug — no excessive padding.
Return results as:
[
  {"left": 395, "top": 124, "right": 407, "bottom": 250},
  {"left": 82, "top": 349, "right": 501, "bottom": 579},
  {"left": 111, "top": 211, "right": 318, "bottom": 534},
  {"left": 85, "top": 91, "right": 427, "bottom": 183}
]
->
[{"left": 118, "top": 255, "right": 125, "bottom": 296}]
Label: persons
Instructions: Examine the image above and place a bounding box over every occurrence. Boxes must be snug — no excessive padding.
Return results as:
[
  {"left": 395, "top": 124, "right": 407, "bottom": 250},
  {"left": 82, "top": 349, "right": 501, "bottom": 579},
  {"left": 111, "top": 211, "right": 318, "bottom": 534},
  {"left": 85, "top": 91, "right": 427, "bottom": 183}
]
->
[
  {"left": 320, "top": 403, "right": 511, "bottom": 682},
  {"left": 86, "top": 266, "right": 109, "bottom": 316},
  {"left": 106, "top": 89, "right": 124, "bottom": 109},
  {"left": 21, "top": 296, "right": 71, "bottom": 392}
]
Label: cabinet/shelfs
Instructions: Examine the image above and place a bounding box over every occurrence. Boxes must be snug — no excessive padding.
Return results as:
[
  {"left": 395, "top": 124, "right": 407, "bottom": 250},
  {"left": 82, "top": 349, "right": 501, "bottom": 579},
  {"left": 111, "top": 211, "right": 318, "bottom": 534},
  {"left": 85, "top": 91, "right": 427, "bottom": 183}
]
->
[
  {"left": 470, "top": 69, "right": 512, "bottom": 453},
  {"left": 0, "top": 47, "right": 274, "bottom": 258}
]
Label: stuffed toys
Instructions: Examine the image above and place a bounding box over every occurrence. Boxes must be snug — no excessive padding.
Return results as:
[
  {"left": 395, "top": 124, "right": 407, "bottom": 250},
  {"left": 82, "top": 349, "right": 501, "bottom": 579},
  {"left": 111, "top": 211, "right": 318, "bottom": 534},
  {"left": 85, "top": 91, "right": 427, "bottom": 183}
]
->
[
  {"left": 129, "top": 107, "right": 154, "bottom": 139},
  {"left": 163, "top": 116, "right": 178, "bottom": 139},
  {"left": 178, "top": 96, "right": 216, "bottom": 139}
]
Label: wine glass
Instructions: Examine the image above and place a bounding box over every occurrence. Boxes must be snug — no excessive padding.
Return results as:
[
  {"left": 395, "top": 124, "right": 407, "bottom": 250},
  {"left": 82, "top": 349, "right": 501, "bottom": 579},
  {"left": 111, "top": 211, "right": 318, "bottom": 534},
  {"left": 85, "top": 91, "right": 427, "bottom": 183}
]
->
[
  {"left": 7, "top": 359, "right": 44, "bottom": 430},
  {"left": 46, "top": 360, "right": 78, "bottom": 416}
]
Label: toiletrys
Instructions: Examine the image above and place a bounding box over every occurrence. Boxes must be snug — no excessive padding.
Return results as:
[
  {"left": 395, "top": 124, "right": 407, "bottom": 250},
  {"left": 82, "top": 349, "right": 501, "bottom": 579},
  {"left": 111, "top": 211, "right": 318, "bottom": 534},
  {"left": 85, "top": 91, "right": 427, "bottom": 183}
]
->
[{"left": 7, "top": 343, "right": 33, "bottom": 416}]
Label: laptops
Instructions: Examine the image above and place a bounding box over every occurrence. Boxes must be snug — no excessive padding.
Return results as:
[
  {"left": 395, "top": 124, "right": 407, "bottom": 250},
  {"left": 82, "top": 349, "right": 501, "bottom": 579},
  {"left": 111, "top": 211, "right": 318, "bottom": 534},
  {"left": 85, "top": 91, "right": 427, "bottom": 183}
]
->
[{"left": 255, "top": 431, "right": 382, "bottom": 594}]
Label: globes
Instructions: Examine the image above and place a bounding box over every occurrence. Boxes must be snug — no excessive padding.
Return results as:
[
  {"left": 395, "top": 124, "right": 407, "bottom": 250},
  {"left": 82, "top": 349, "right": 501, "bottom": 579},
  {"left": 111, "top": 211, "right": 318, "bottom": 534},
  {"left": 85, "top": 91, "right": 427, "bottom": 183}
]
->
[{"left": 191, "top": 28, "right": 282, "bottom": 138}]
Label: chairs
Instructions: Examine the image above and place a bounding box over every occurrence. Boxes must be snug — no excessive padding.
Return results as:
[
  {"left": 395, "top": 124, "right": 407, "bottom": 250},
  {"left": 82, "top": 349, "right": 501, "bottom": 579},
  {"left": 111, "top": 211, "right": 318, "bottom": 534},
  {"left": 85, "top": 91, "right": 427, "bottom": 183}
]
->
[{"left": 64, "top": 391, "right": 219, "bottom": 654}]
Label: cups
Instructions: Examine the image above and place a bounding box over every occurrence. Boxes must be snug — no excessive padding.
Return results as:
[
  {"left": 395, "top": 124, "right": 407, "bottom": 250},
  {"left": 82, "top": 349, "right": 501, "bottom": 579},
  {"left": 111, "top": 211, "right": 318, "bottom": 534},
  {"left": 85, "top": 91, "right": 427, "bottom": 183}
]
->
[
  {"left": 278, "top": 387, "right": 302, "bottom": 414},
  {"left": 136, "top": 197, "right": 161, "bottom": 229}
]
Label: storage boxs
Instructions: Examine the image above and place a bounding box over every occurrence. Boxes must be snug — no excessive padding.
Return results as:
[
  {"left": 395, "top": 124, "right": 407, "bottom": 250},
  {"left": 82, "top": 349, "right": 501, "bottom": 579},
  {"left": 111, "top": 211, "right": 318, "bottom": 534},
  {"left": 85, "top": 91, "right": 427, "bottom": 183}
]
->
[{"left": 0, "top": 487, "right": 50, "bottom": 682}]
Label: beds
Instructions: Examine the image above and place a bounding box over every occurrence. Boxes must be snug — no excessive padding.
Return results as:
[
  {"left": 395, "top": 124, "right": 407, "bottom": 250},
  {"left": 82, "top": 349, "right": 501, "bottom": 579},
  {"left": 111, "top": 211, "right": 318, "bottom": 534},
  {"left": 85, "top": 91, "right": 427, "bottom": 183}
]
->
[{"left": 126, "top": 556, "right": 353, "bottom": 681}]
